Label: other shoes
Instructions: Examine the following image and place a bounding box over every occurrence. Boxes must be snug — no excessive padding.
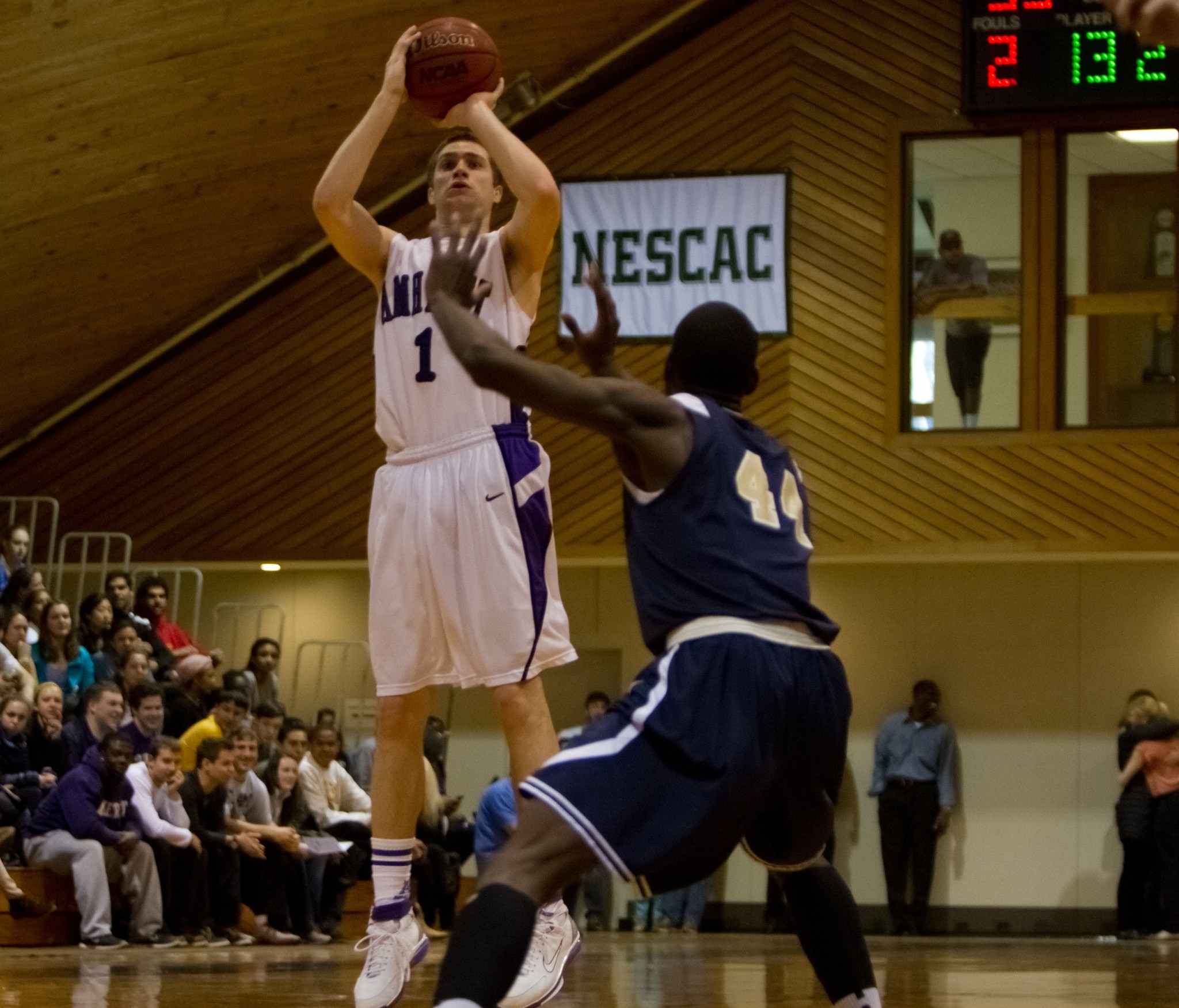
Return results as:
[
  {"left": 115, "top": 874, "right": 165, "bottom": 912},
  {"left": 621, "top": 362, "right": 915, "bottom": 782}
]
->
[
  {"left": 260, "top": 925, "right": 301, "bottom": 944},
  {"left": 10, "top": 895, "right": 57, "bottom": 916},
  {"left": 200, "top": 926, "right": 231, "bottom": 945},
  {"left": 186, "top": 934, "right": 207, "bottom": 946},
  {"left": 306, "top": 930, "right": 332, "bottom": 943},
  {"left": 222, "top": 926, "right": 255, "bottom": 944}
]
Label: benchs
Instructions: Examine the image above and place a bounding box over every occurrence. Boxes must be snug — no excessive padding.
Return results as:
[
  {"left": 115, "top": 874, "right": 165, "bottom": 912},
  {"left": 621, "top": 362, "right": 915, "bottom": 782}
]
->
[{"left": 0, "top": 864, "right": 373, "bottom": 947}]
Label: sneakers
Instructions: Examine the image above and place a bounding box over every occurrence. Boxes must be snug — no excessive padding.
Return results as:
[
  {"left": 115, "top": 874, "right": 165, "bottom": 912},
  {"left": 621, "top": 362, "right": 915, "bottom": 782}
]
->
[
  {"left": 354, "top": 906, "right": 429, "bottom": 1008},
  {"left": 127, "top": 933, "right": 180, "bottom": 947},
  {"left": 79, "top": 935, "right": 128, "bottom": 950},
  {"left": 496, "top": 899, "right": 582, "bottom": 1008}
]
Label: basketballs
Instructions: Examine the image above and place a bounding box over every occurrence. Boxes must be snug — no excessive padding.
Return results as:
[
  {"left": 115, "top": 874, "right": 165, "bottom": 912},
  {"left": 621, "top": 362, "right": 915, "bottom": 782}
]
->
[{"left": 400, "top": 16, "right": 500, "bottom": 117}]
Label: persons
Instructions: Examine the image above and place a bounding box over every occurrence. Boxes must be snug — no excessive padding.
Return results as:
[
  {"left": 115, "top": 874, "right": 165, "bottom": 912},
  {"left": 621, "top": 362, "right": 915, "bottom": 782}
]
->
[
  {"left": 1114, "top": 686, "right": 1179, "bottom": 940},
  {"left": 915, "top": 227, "right": 995, "bottom": 429},
  {"left": 423, "top": 194, "right": 887, "bottom": 1008},
  {"left": 0, "top": 522, "right": 519, "bottom": 953},
  {"left": 311, "top": 20, "right": 585, "bottom": 1008},
  {"left": 559, "top": 687, "right": 707, "bottom": 933},
  {"left": 22, "top": 730, "right": 181, "bottom": 953},
  {"left": 865, "top": 676, "right": 959, "bottom": 937}
]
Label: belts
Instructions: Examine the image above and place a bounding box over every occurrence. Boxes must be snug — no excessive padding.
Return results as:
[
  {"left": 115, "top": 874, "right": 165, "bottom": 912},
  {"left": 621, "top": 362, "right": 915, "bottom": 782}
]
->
[{"left": 889, "top": 779, "right": 937, "bottom": 787}]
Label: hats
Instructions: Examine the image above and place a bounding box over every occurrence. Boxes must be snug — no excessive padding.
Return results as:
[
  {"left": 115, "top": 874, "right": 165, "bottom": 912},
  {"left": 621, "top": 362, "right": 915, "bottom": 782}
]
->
[{"left": 177, "top": 653, "right": 212, "bottom": 684}]
